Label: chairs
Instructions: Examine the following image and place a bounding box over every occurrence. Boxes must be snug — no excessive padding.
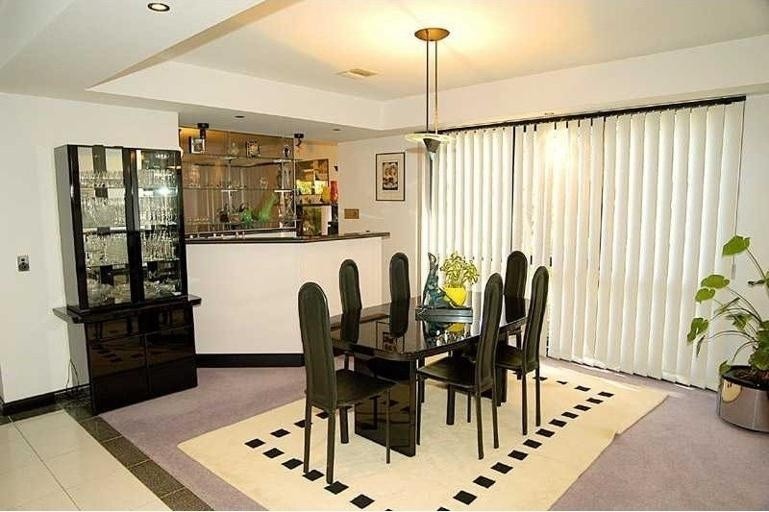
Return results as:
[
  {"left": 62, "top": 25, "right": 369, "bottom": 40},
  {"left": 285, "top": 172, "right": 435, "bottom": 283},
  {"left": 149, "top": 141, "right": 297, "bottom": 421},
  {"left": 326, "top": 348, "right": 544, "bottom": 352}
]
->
[
  {"left": 495, "top": 251, "right": 549, "bottom": 437},
  {"left": 416, "top": 272, "right": 503, "bottom": 460},
  {"left": 338, "top": 258, "right": 363, "bottom": 369},
  {"left": 298, "top": 281, "right": 397, "bottom": 483},
  {"left": 388, "top": 251, "right": 425, "bottom": 370}
]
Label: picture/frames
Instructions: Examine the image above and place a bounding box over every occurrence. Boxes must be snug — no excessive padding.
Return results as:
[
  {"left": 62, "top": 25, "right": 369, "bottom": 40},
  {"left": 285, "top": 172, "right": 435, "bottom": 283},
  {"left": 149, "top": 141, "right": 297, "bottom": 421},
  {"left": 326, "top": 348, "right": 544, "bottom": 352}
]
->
[{"left": 376, "top": 152, "right": 405, "bottom": 201}]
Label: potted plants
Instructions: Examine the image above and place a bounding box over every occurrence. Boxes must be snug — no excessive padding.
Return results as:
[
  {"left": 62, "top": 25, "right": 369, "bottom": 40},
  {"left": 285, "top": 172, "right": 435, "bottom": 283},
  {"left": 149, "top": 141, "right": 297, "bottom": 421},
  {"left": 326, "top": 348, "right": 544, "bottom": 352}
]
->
[{"left": 687, "top": 235, "right": 769, "bottom": 433}]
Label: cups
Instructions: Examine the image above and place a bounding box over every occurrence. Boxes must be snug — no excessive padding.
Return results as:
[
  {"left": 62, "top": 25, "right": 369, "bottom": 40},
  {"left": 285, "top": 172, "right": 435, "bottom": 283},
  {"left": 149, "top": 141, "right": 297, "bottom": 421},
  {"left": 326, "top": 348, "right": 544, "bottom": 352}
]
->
[{"left": 259, "top": 177, "right": 268, "bottom": 189}]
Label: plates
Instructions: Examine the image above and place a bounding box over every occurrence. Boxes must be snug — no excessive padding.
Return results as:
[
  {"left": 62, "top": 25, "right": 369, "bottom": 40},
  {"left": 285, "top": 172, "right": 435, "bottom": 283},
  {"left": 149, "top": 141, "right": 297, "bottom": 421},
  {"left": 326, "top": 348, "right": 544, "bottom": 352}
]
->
[{"left": 114, "top": 263, "right": 178, "bottom": 301}]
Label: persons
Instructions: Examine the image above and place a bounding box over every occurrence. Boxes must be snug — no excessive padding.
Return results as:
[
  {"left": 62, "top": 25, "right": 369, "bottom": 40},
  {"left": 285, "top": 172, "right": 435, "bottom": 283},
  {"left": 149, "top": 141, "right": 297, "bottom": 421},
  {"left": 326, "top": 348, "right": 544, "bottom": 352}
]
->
[{"left": 383, "top": 163, "right": 398, "bottom": 190}]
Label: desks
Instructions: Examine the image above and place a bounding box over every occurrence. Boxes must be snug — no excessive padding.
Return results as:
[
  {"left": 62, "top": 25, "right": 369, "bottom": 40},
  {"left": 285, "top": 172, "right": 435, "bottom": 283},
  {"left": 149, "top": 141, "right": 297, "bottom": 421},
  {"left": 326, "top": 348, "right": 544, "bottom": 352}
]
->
[{"left": 328, "top": 289, "right": 534, "bottom": 459}]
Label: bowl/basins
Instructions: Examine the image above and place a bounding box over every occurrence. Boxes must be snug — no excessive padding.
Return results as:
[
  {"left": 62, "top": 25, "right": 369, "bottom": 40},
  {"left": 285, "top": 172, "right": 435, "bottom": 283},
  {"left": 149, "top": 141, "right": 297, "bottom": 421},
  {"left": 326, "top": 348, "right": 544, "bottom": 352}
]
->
[{"left": 86, "top": 270, "right": 112, "bottom": 305}]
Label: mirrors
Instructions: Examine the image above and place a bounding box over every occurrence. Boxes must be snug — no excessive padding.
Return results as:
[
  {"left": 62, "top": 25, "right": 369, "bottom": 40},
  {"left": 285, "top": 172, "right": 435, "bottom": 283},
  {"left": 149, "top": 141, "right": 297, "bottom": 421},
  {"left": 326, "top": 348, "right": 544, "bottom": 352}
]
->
[{"left": 295, "top": 159, "right": 329, "bottom": 206}]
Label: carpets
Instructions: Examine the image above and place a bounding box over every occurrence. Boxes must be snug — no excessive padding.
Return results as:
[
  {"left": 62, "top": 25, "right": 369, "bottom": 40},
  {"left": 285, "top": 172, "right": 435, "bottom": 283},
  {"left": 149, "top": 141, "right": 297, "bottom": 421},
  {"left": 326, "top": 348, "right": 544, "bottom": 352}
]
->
[{"left": 176, "top": 353, "right": 670, "bottom": 512}]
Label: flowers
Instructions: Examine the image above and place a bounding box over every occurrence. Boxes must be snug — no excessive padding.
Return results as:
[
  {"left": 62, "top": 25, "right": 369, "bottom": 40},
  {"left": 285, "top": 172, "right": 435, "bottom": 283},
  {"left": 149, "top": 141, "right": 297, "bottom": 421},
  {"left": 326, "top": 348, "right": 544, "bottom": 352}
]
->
[{"left": 439, "top": 253, "right": 480, "bottom": 287}]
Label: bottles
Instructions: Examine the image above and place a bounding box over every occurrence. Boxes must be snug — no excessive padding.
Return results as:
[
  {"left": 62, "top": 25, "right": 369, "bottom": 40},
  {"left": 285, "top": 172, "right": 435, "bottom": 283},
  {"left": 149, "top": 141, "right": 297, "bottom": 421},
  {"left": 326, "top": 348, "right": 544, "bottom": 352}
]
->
[
  {"left": 321, "top": 185, "right": 331, "bottom": 203},
  {"left": 421, "top": 320, "right": 439, "bottom": 350},
  {"left": 330, "top": 180, "right": 339, "bottom": 203},
  {"left": 227, "top": 141, "right": 240, "bottom": 156},
  {"left": 423, "top": 249, "right": 439, "bottom": 307}
]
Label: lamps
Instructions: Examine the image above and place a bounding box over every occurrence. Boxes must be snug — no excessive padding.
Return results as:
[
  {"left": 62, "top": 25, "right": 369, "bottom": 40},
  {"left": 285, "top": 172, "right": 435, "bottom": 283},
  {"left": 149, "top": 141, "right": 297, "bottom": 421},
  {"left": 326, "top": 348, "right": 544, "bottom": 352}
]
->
[{"left": 404, "top": 27, "right": 457, "bottom": 160}]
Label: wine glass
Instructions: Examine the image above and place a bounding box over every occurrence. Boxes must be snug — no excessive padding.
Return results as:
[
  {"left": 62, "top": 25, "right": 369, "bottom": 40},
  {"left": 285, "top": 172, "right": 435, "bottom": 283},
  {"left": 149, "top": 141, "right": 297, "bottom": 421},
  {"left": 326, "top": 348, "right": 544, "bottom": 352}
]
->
[
  {"left": 84, "top": 231, "right": 174, "bottom": 265},
  {"left": 81, "top": 194, "right": 174, "bottom": 231},
  {"left": 80, "top": 169, "right": 173, "bottom": 187}
]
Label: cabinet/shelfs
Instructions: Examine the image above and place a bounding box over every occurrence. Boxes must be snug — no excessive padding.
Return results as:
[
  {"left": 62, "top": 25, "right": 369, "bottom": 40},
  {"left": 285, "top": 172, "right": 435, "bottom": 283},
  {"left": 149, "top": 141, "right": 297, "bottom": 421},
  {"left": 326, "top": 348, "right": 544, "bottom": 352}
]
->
[
  {"left": 180, "top": 125, "right": 297, "bottom": 239},
  {"left": 52, "top": 144, "right": 201, "bottom": 417}
]
